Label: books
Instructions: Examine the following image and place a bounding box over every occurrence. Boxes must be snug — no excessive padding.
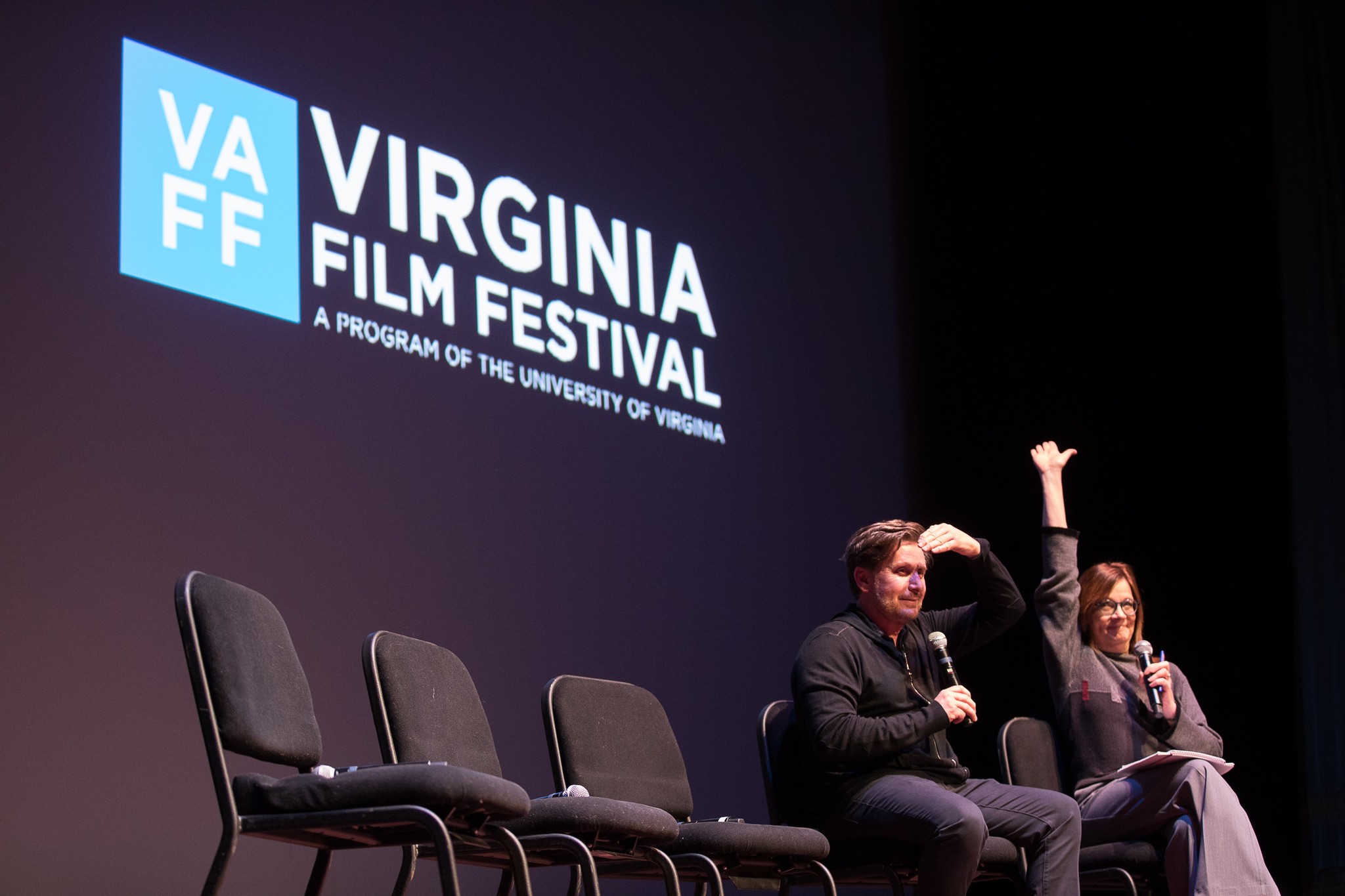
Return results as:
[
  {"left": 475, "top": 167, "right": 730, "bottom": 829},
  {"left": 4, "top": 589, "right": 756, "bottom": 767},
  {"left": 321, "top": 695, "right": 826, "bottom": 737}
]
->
[{"left": 1117, "top": 750, "right": 1235, "bottom": 777}]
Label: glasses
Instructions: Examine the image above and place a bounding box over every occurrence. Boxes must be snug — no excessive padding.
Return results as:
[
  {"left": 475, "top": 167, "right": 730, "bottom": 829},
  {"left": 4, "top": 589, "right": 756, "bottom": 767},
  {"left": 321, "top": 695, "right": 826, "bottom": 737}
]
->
[{"left": 1092, "top": 598, "right": 1140, "bottom": 615}]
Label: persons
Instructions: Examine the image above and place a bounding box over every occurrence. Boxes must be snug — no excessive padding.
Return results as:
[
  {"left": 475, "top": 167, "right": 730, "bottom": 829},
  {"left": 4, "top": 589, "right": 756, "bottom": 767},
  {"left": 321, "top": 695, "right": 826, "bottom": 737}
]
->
[
  {"left": 790, "top": 520, "right": 1084, "bottom": 896},
  {"left": 1031, "top": 441, "right": 1288, "bottom": 896}
]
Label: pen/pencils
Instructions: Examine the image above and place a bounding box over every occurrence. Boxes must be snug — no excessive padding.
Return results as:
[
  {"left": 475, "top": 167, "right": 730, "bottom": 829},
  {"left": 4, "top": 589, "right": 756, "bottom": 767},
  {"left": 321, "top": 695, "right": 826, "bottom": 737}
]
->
[{"left": 1159, "top": 649, "right": 1166, "bottom": 692}]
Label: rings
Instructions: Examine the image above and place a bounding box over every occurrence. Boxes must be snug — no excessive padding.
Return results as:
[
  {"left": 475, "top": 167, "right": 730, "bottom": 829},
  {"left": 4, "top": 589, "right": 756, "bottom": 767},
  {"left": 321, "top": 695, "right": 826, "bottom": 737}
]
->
[{"left": 1166, "top": 672, "right": 1171, "bottom": 679}]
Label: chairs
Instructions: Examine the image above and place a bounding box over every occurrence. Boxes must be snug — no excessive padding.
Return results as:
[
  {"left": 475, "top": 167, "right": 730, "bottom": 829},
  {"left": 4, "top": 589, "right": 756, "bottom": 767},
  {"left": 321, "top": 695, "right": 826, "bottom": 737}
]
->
[{"left": 175, "top": 570, "right": 1159, "bottom": 896}]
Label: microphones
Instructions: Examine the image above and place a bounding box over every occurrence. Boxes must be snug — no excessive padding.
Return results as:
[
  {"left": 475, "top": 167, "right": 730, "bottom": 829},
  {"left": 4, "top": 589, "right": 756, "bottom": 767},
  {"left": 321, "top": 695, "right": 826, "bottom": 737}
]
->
[
  {"left": 311, "top": 765, "right": 360, "bottom": 778},
  {"left": 927, "top": 631, "right": 961, "bottom": 688},
  {"left": 677, "top": 815, "right": 742, "bottom": 824},
  {"left": 1133, "top": 640, "right": 1164, "bottom": 719},
  {"left": 535, "top": 784, "right": 591, "bottom": 799}
]
